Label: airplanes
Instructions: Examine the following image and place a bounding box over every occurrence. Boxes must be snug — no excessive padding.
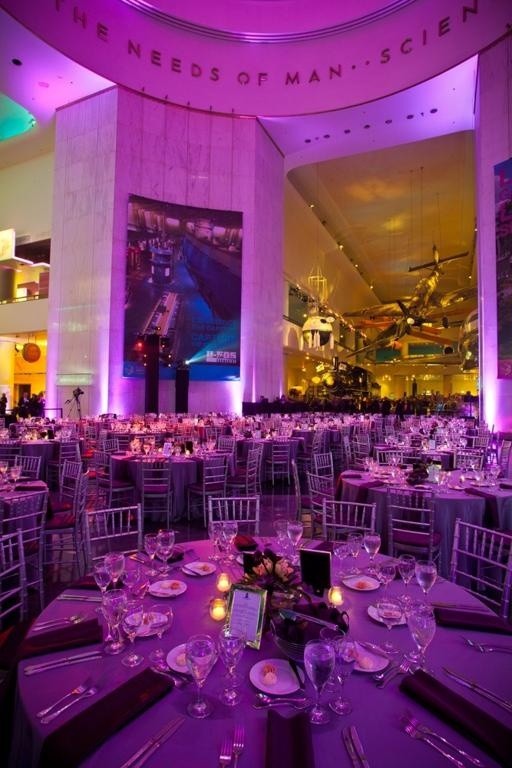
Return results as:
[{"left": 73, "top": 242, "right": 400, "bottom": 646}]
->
[{"left": 334, "top": 247, "right": 480, "bottom": 370}]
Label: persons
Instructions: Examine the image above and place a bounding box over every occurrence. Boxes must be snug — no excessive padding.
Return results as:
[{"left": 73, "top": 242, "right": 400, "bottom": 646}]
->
[
  {"left": 0, "top": 392, "right": 7, "bottom": 412},
  {"left": 18, "top": 392, "right": 30, "bottom": 406},
  {"left": 464, "top": 390, "right": 474, "bottom": 402},
  {"left": 370, "top": 397, "right": 402, "bottom": 416}
]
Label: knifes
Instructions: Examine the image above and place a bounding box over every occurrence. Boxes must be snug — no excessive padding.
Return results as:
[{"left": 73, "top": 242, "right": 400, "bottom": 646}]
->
[
  {"left": 20, "top": 592, "right": 103, "bottom": 676},
  {"left": 348, "top": 725, "right": 371, "bottom": 768},
  {"left": 430, "top": 597, "right": 511, "bottom": 714},
  {"left": 120, "top": 714, "right": 181, "bottom": 768},
  {"left": 130, "top": 720, "right": 186, "bottom": 767},
  {"left": 341, "top": 726, "right": 362, "bottom": 768}
]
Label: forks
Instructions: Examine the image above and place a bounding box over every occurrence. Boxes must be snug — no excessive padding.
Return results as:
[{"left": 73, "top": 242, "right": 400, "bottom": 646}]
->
[
  {"left": 218, "top": 735, "right": 232, "bottom": 768},
  {"left": 34, "top": 678, "right": 91, "bottom": 718},
  {"left": 398, "top": 720, "right": 465, "bottom": 768},
  {"left": 232, "top": 726, "right": 247, "bottom": 768},
  {"left": 409, "top": 717, "right": 485, "bottom": 768}
]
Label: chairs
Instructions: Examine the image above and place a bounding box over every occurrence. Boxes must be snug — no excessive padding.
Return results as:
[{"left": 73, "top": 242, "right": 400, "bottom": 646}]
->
[{"left": 0, "top": 409, "right": 512, "bottom": 671}]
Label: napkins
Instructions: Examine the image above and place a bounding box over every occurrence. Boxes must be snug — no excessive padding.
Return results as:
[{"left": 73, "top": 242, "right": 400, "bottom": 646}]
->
[
  {"left": 264, "top": 700, "right": 314, "bottom": 768},
  {"left": 36, "top": 664, "right": 176, "bottom": 768},
  {"left": 399, "top": 668, "right": 512, "bottom": 767}
]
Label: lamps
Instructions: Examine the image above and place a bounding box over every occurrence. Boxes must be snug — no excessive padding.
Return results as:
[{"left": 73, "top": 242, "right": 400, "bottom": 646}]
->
[
  {"left": 408, "top": 163, "right": 471, "bottom": 273},
  {"left": 297, "top": 161, "right": 337, "bottom": 352}
]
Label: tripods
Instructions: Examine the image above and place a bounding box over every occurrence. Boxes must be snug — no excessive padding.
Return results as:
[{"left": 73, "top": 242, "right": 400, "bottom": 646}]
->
[{"left": 64, "top": 396, "right": 81, "bottom": 419}]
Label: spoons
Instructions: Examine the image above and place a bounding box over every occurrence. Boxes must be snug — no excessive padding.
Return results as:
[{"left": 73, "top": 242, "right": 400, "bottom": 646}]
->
[
  {"left": 41, "top": 684, "right": 98, "bottom": 724},
  {"left": 250, "top": 689, "right": 308, "bottom": 711}
]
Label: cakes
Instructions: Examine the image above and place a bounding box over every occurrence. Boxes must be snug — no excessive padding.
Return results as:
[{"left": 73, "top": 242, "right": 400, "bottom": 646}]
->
[
  {"left": 176, "top": 654, "right": 188, "bottom": 665},
  {"left": 193, "top": 563, "right": 210, "bottom": 572},
  {"left": 358, "top": 658, "right": 373, "bottom": 670},
  {"left": 143, "top": 614, "right": 154, "bottom": 624},
  {"left": 161, "top": 581, "right": 180, "bottom": 589},
  {"left": 262, "top": 665, "right": 276, "bottom": 686},
  {"left": 355, "top": 581, "right": 372, "bottom": 589}
]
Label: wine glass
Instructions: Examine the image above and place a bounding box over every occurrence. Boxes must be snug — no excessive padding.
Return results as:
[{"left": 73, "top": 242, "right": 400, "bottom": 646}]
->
[
  {"left": 215, "top": 627, "right": 247, "bottom": 708},
  {"left": 329, "top": 532, "right": 348, "bottom": 580},
  {"left": 289, "top": 523, "right": 304, "bottom": 563},
  {"left": 101, "top": 588, "right": 126, "bottom": 655},
  {"left": 404, "top": 604, "right": 439, "bottom": 672},
  {"left": 329, "top": 640, "right": 354, "bottom": 717},
  {"left": 304, "top": 413, "right": 505, "bottom": 493},
  {"left": 153, "top": 527, "right": 175, "bottom": 579},
  {"left": 377, "top": 558, "right": 396, "bottom": 600},
  {"left": 348, "top": 532, "right": 364, "bottom": 573},
  {"left": 339, "top": 575, "right": 379, "bottom": 592},
  {"left": 103, "top": 554, "right": 126, "bottom": 598},
  {"left": 364, "top": 531, "right": 381, "bottom": 573},
  {"left": 86, "top": 413, "right": 304, "bottom": 461},
  {"left": 221, "top": 521, "right": 239, "bottom": 559},
  {"left": 377, "top": 594, "right": 399, "bottom": 658},
  {"left": 205, "top": 520, "right": 223, "bottom": 563},
  {"left": 185, "top": 634, "right": 217, "bottom": 721},
  {"left": 302, "top": 640, "right": 332, "bottom": 726},
  {"left": 0, "top": 459, "right": 26, "bottom": 494},
  {"left": 1, "top": 416, "right": 75, "bottom": 442},
  {"left": 414, "top": 559, "right": 438, "bottom": 603},
  {"left": 117, "top": 600, "right": 145, "bottom": 669},
  {"left": 272, "top": 520, "right": 289, "bottom": 556},
  {"left": 140, "top": 532, "right": 159, "bottom": 580},
  {"left": 396, "top": 558, "right": 416, "bottom": 603},
  {"left": 144, "top": 604, "right": 173, "bottom": 667},
  {"left": 94, "top": 561, "right": 112, "bottom": 600}
]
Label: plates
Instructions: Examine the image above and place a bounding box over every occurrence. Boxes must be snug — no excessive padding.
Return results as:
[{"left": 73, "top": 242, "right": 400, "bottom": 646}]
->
[
  {"left": 165, "top": 642, "right": 217, "bottom": 677},
  {"left": 147, "top": 579, "right": 187, "bottom": 600},
  {"left": 367, "top": 599, "right": 410, "bottom": 625},
  {"left": 338, "top": 637, "right": 389, "bottom": 675},
  {"left": 123, "top": 609, "right": 167, "bottom": 640},
  {"left": 249, "top": 656, "right": 305, "bottom": 696},
  {"left": 178, "top": 559, "right": 217, "bottom": 578}
]
